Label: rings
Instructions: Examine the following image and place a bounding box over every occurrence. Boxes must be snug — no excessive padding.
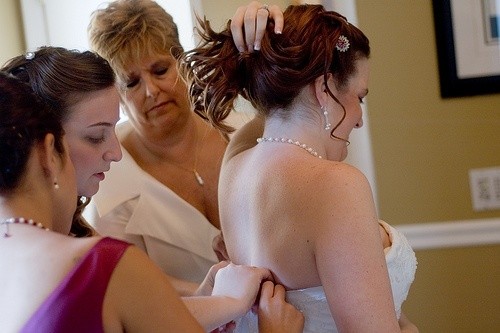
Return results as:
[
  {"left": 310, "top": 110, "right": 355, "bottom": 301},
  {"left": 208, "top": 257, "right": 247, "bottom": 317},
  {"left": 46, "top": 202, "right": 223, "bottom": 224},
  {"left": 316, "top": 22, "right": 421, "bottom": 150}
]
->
[{"left": 259, "top": 3, "right": 271, "bottom": 18}]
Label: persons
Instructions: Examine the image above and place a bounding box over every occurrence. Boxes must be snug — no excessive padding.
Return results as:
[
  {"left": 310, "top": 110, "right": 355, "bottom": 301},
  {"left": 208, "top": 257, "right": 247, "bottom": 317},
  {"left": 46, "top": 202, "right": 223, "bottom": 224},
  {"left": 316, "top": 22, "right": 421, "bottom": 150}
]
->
[
  {"left": 0, "top": 46, "right": 273, "bottom": 333},
  {"left": 81, "top": 0, "right": 284, "bottom": 284},
  {"left": 0, "top": 70, "right": 305, "bottom": 333},
  {"left": 180, "top": 0, "right": 419, "bottom": 333}
]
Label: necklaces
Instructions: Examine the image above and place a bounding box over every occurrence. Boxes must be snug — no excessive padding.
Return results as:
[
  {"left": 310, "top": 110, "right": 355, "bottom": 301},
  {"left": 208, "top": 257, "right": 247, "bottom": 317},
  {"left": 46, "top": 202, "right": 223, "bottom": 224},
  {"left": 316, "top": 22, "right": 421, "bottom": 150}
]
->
[
  {"left": 255, "top": 136, "right": 322, "bottom": 159},
  {"left": 0, "top": 217, "right": 50, "bottom": 237},
  {"left": 149, "top": 120, "right": 204, "bottom": 187}
]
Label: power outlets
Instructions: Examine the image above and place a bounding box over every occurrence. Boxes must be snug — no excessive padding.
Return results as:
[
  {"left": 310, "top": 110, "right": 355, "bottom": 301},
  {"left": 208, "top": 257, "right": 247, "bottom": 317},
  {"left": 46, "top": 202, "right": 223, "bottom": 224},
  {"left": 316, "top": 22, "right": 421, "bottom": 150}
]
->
[{"left": 470, "top": 166, "right": 500, "bottom": 209}]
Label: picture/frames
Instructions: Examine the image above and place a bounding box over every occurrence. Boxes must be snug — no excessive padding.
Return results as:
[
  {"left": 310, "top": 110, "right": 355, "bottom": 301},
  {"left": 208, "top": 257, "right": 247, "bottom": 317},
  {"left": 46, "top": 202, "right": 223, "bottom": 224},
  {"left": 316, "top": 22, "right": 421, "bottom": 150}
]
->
[{"left": 432, "top": 0, "right": 500, "bottom": 97}]
